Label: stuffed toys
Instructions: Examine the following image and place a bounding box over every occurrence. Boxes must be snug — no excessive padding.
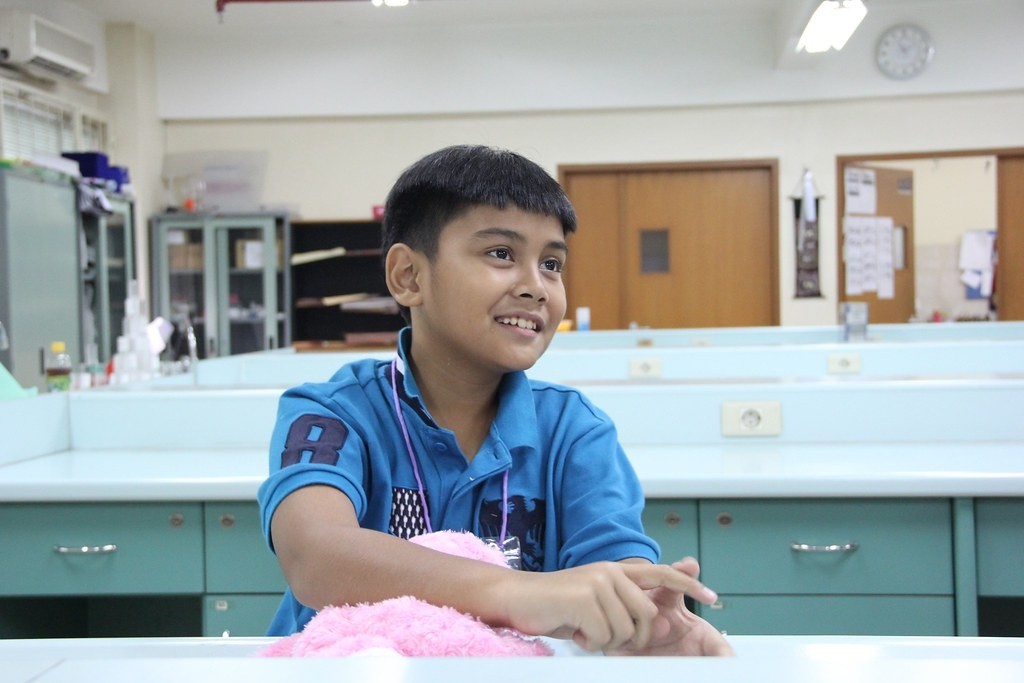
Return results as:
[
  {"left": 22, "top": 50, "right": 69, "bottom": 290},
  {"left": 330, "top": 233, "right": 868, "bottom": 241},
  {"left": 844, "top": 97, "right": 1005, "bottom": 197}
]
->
[{"left": 252, "top": 531, "right": 555, "bottom": 658}]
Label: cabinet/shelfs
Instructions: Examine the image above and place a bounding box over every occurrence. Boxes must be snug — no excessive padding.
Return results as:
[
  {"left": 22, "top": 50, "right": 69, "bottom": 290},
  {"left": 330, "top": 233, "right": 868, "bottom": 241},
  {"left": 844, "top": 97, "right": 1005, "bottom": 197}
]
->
[
  {"left": 151, "top": 212, "right": 277, "bottom": 361},
  {"left": 1, "top": 172, "right": 137, "bottom": 387},
  {"left": 283, "top": 211, "right": 412, "bottom": 345},
  {"left": 0, "top": 320, "right": 1023, "bottom": 637}
]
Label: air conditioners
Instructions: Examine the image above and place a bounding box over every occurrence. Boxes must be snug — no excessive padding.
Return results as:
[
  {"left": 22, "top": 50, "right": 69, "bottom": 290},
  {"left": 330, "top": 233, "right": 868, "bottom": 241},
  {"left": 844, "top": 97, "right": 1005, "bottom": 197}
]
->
[{"left": 1, "top": 14, "right": 95, "bottom": 83}]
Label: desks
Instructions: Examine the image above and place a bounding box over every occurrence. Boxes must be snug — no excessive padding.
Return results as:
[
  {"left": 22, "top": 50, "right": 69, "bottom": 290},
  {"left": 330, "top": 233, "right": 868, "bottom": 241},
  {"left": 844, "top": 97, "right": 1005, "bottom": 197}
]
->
[{"left": 1, "top": 636, "right": 1024, "bottom": 683}]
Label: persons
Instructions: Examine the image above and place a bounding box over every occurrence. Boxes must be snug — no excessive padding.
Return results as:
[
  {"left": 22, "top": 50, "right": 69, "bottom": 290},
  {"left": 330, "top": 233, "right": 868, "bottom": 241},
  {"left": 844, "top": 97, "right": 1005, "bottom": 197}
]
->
[{"left": 258, "top": 145, "right": 736, "bottom": 657}]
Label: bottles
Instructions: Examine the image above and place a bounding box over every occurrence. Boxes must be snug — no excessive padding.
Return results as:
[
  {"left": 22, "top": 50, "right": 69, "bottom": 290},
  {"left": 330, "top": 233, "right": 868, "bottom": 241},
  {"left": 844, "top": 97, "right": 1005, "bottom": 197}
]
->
[{"left": 47, "top": 342, "right": 72, "bottom": 393}]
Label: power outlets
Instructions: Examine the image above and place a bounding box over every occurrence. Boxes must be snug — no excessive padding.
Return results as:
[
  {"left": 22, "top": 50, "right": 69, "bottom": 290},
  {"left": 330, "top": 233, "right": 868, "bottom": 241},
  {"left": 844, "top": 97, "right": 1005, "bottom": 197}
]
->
[{"left": 722, "top": 399, "right": 782, "bottom": 436}]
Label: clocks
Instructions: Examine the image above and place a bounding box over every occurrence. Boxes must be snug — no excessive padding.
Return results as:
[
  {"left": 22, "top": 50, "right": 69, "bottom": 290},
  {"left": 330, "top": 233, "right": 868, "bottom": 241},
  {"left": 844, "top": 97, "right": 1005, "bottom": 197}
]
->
[{"left": 875, "top": 22, "right": 933, "bottom": 80}]
